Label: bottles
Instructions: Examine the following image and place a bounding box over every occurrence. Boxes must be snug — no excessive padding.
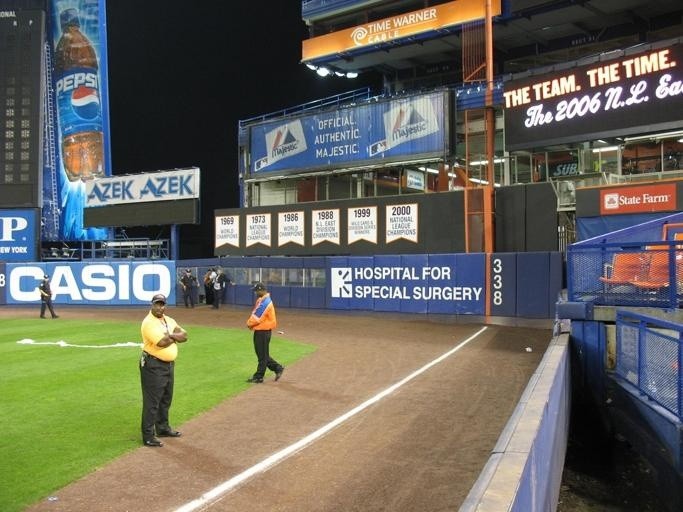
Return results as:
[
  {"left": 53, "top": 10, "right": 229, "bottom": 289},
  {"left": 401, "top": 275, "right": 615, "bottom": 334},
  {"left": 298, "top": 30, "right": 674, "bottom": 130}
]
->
[{"left": 53, "top": 6, "right": 106, "bottom": 181}]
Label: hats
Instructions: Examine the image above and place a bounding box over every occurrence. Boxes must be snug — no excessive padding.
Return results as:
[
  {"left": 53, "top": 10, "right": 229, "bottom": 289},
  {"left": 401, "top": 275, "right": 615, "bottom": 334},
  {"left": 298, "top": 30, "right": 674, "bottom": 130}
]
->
[
  {"left": 249, "top": 283, "right": 265, "bottom": 290},
  {"left": 152, "top": 294, "right": 167, "bottom": 304}
]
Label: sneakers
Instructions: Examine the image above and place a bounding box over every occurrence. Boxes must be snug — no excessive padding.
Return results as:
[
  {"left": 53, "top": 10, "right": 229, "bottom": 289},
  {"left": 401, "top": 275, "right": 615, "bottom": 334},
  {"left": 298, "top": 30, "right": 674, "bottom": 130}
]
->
[
  {"left": 143, "top": 436, "right": 163, "bottom": 447},
  {"left": 158, "top": 427, "right": 182, "bottom": 438},
  {"left": 184, "top": 302, "right": 221, "bottom": 309},
  {"left": 39, "top": 313, "right": 60, "bottom": 319},
  {"left": 274, "top": 364, "right": 285, "bottom": 381},
  {"left": 246, "top": 375, "right": 264, "bottom": 383}
]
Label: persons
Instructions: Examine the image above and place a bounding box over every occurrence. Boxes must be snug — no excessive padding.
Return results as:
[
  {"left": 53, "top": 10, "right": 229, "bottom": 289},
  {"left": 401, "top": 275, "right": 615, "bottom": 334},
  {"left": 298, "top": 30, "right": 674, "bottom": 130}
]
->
[
  {"left": 39, "top": 275, "right": 60, "bottom": 318},
  {"left": 246, "top": 283, "right": 284, "bottom": 383},
  {"left": 139, "top": 294, "right": 187, "bottom": 447},
  {"left": 179, "top": 266, "right": 230, "bottom": 309}
]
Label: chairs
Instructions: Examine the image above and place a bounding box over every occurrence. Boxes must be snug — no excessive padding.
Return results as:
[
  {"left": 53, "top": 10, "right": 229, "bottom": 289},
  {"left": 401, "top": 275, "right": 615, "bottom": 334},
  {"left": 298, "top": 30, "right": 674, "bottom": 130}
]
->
[{"left": 600, "top": 233, "right": 683, "bottom": 296}]
